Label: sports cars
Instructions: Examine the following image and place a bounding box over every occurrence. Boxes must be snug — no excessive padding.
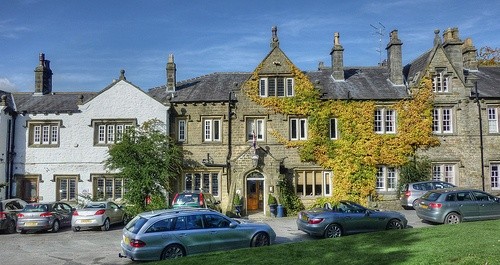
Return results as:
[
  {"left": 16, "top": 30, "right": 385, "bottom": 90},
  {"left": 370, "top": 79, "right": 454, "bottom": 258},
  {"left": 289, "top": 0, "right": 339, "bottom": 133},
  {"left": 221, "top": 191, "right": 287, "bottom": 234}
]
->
[{"left": 296, "top": 200, "right": 409, "bottom": 238}]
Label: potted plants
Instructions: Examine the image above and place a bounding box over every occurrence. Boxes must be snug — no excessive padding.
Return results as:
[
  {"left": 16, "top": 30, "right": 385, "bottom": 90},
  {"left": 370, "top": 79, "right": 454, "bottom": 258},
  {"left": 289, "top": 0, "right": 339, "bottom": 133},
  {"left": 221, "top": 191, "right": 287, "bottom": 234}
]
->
[
  {"left": 233, "top": 194, "right": 243, "bottom": 216},
  {"left": 267, "top": 194, "right": 277, "bottom": 218}
]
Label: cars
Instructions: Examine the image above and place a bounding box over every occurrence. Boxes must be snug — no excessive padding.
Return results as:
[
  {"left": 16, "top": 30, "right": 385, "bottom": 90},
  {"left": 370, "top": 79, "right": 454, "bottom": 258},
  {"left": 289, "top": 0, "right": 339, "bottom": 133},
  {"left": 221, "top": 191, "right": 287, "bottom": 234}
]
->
[
  {"left": 416, "top": 187, "right": 500, "bottom": 224},
  {"left": 70, "top": 200, "right": 128, "bottom": 232},
  {"left": 15, "top": 201, "right": 74, "bottom": 235}
]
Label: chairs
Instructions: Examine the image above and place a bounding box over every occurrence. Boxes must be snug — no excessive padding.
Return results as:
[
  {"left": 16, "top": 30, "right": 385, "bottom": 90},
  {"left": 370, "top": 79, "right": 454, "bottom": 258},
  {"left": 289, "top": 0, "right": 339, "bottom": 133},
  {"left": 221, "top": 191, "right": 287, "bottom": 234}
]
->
[
  {"left": 324, "top": 203, "right": 331, "bottom": 209},
  {"left": 333, "top": 205, "right": 341, "bottom": 211}
]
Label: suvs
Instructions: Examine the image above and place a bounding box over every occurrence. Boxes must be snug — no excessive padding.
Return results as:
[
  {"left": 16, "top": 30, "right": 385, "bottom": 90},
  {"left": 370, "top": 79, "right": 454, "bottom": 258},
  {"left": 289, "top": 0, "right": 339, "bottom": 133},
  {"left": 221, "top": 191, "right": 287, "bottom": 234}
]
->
[
  {"left": 118, "top": 207, "right": 277, "bottom": 261},
  {"left": 172, "top": 190, "right": 223, "bottom": 212},
  {"left": 400, "top": 180, "right": 457, "bottom": 210},
  {"left": 0, "top": 198, "right": 28, "bottom": 234}
]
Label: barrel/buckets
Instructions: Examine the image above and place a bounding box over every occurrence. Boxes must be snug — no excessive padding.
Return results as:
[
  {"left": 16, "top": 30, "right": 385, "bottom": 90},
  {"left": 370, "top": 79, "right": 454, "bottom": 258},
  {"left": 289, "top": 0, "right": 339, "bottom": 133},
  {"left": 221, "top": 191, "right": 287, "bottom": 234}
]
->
[{"left": 277, "top": 204, "right": 287, "bottom": 219}]
